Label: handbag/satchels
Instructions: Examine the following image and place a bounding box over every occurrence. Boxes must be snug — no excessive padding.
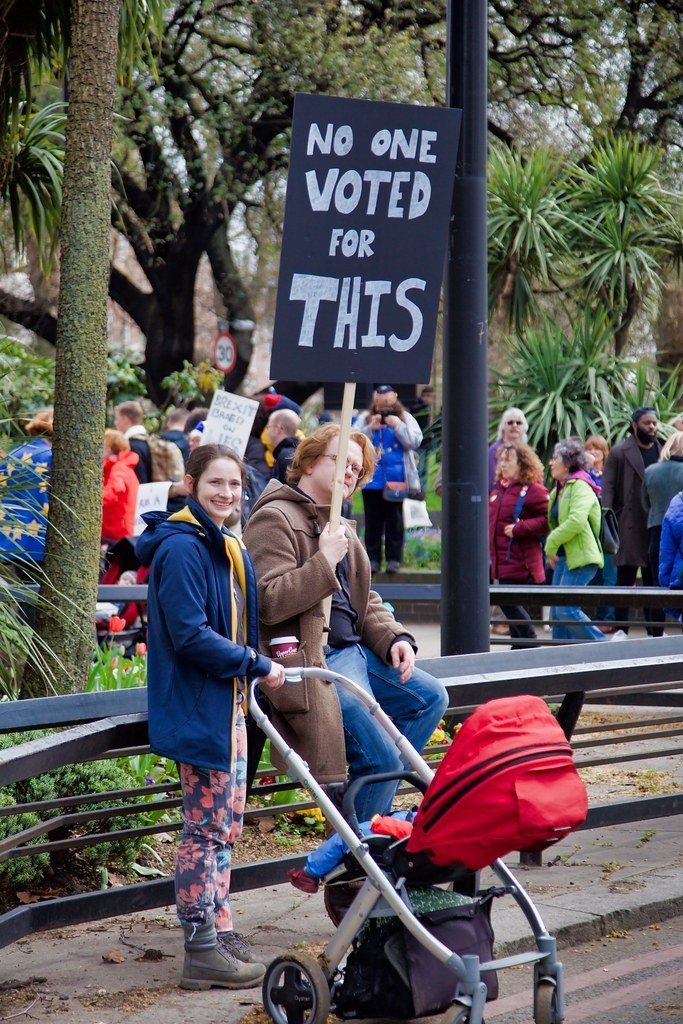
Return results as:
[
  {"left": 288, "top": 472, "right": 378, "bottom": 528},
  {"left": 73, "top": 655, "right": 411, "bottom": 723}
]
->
[{"left": 383, "top": 481, "right": 407, "bottom": 501}]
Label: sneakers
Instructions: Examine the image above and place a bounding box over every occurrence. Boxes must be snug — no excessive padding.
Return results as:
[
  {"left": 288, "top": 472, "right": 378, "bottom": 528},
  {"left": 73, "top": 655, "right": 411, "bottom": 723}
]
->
[
  {"left": 180, "top": 940, "right": 267, "bottom": 990},
  {"left": 287, "top": 866, "right": 319, "bottom": 893},
  {"left": 216, "top": 930, "right": 276, "bottom": 969}
]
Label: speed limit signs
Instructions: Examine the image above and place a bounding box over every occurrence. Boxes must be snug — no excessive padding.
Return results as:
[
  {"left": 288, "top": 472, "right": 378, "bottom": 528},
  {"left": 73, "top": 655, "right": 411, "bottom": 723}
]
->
[{"left": 214, "top": 333, "right": 237, "bottom": 373}]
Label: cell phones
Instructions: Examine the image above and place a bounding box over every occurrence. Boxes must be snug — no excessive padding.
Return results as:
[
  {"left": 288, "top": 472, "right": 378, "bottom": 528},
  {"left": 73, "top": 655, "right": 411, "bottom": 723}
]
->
[{"left": 380, "top": 410, "right": 390, "bottom": 424}]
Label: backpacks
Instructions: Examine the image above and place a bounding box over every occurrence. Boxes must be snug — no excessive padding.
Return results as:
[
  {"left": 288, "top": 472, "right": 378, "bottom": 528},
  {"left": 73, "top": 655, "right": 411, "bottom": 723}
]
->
[
  {"left": 571, "top": 480, "right": 620, "bottom": 556},
  {"left": 129, "top": 434, "right": 185, "bottom": 483}
]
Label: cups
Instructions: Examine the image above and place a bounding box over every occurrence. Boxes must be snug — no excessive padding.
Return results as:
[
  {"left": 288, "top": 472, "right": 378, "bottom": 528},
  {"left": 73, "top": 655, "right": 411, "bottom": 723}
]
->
[{"left": 268, "top": 636, "right": 300, "bottom": 658}]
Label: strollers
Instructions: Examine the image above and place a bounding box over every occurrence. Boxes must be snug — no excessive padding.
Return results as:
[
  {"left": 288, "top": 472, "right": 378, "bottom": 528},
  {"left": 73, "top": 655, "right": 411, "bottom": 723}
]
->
[{"left": 240, "top": 664, "right": 598, "bottom": 1023}]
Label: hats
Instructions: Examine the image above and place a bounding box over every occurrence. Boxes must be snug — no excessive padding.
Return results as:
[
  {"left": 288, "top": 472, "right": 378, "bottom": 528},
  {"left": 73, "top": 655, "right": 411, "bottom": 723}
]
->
[
  {"left": 372, "top": 385, "right": 398, "bottom": 407},
  {"left": 627, "top": 406, "right": 655, "bottom": 434}
]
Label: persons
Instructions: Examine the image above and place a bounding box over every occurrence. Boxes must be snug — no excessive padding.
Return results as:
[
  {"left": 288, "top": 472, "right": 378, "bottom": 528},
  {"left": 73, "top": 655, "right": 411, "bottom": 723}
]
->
[
  {"left": 134, "top": 443, "right": 286, "bottom": 991},
  {"left": 287, "top": 804, "right": 418, "bottom": 893},
  {"left": 243, "top": 423, "right": 449, "bottom": 839},
  {"left": 488, "top": 408, "right": 683, "bottom": 650},
  {"left": 0, "top": 384, "right": 437, "bottom": 631}
]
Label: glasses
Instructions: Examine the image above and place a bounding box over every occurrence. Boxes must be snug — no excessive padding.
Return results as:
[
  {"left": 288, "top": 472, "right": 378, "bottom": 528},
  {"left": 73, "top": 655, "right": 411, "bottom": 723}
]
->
[
  {"left": 321, "top": 454, "right": 365, "bottom": 479},
  {"left": 506, "top": 420, "right": 523, "bottom": 425},
  {"left": 266, "top": 424, "right": 277, "bottom": 431}
]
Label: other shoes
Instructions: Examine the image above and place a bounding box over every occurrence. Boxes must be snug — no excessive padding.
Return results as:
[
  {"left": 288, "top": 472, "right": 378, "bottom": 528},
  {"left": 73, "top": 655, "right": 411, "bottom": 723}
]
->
[
  {"left": 386, "top": 560, "right": 400, "bottom": 573},
  {"left": 599, "top": 625, "right": 617, "bottom": 633},
  {"left": 611, "top": 629, "right": 627, "bottom": 641},
  {"left": 492, "top": 624, "right": 511, "bottom": 634},
  {"left": 371, "top": 560, "right": 381, "bottom": 573},
  {"left": 319, "top": 780, "right": 346, "bottom": 842}
]
